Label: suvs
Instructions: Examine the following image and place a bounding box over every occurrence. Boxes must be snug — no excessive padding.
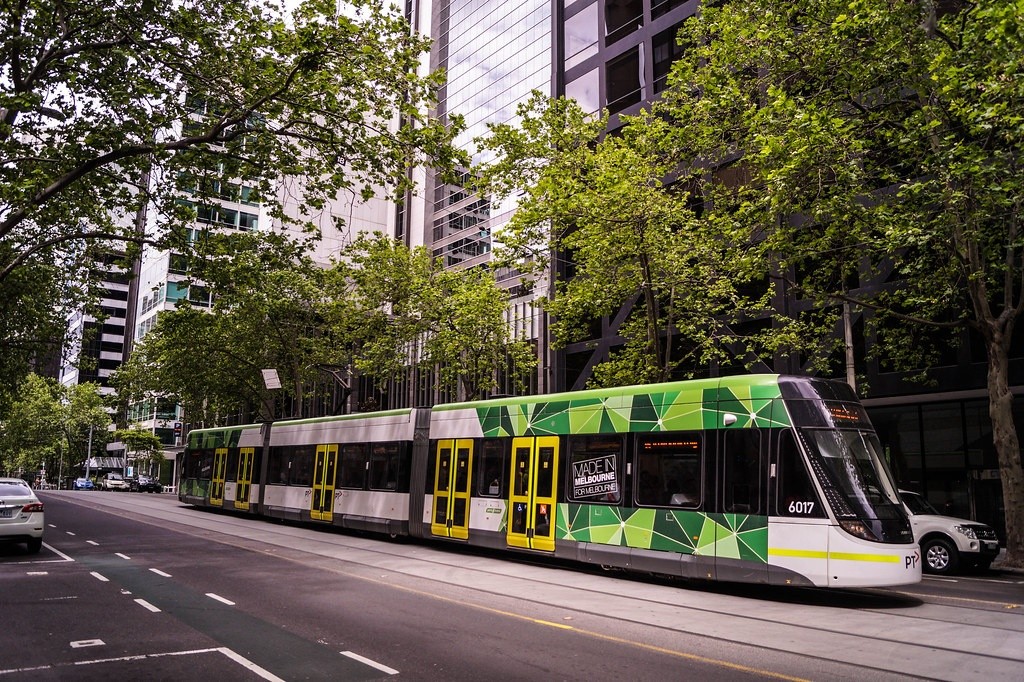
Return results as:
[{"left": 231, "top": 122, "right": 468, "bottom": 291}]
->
[{"left": 860, "top": 485, "right": 1000, "bottom": 573}]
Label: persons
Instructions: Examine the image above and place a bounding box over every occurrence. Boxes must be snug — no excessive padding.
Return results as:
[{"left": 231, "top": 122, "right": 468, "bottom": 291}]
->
[{"left": 35, "top": 477, "right": 42, "bottom": 490}]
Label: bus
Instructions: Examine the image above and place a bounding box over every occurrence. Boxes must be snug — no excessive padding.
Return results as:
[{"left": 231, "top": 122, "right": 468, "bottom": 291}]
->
[
  {"left": 21, "top": 471, "right": 49, "bottom": 482},
  {"left": 178, "top": 373, "right": 923, "bottom": 588},
  {"left": 856, "top": 387, "right": 1024, "bottom": 558}
]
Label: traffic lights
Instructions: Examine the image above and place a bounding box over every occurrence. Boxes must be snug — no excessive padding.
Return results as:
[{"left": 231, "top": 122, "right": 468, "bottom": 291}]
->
[{"left": 174, "top": 423, "right": 181, "bottom": 437}]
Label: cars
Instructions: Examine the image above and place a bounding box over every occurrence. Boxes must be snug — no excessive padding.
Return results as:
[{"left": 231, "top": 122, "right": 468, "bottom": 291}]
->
[
  {"left": 101, "top": 471, "right": 126, "bottom": 491},
  {"left": 130, "top": 475, "right": 161, "bottom": 493},
  {"left": 0, "top": 478, "right": 44, "bottom": 552},
  {"left": 73, "top": 478, "right": 93, "bottom": 490}
]
